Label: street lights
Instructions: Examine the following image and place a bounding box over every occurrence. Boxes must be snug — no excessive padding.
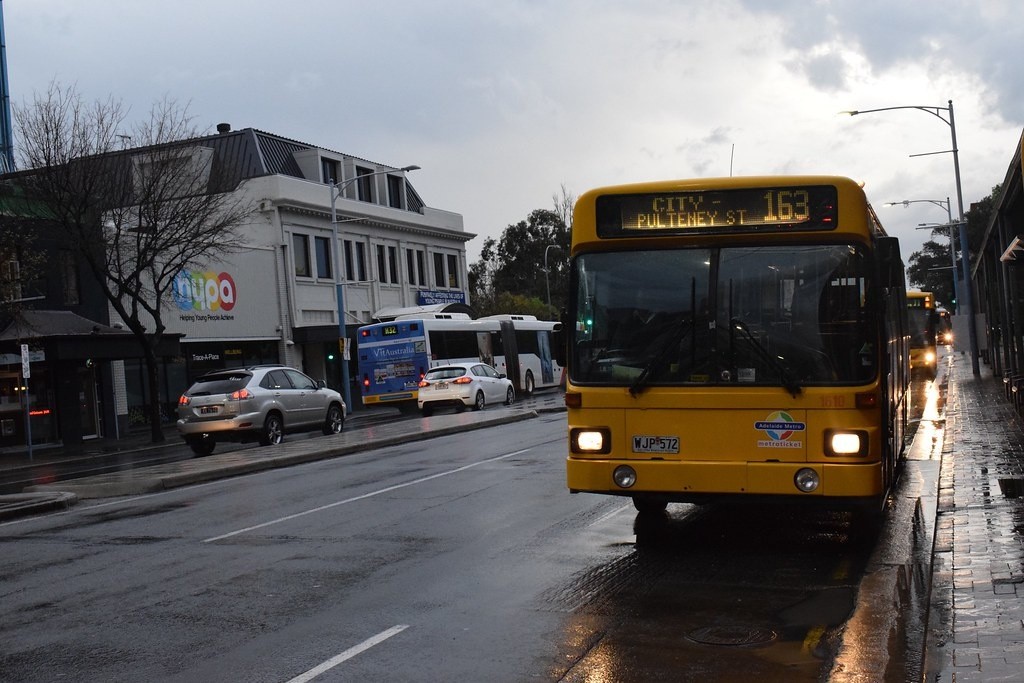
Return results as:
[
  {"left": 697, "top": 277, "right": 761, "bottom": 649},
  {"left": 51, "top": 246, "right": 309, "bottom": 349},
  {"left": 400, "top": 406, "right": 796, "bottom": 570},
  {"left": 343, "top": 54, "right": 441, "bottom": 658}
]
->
[
  {"left": 849, "top": 99, "right": 980, "bottom": 376},
  {"left": 545, "top": 244, "right": 561, "bottom": 321},
  {"left": 918, "top": 222, "right": 961, "bottom": 314},
  {"left": 890, "top": 197, "right": 961, "bottom": 315},
  {"left": 328, "top": 164, "right": 422, "bottom": 413}
]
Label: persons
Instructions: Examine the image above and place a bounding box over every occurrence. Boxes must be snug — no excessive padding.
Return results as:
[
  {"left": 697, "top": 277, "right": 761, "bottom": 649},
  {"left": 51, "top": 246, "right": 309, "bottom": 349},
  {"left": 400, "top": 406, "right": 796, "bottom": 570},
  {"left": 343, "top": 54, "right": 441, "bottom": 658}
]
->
[{"left": 609, "top": 277, "right": 689, "bottom": 375}]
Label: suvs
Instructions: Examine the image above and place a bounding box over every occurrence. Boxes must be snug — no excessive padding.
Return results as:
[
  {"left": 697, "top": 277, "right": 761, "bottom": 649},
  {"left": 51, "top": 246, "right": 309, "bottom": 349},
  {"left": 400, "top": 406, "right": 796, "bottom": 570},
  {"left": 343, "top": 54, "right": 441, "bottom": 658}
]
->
[{"left": 176, "top": 364, "right": 347, "bottom": 455}]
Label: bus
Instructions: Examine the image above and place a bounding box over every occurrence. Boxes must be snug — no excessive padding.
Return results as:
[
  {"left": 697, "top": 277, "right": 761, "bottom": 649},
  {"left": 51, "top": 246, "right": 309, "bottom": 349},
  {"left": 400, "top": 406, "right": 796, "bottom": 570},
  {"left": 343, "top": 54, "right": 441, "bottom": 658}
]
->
[
  {"left": 562, "top": 175, "right": 912, "bottom": 512},
  {"left": 357, "top": 312, "right": 563, "bottom": 412},
  {"left": 906, "top": 289, "right": 952, "bottom": 377}
]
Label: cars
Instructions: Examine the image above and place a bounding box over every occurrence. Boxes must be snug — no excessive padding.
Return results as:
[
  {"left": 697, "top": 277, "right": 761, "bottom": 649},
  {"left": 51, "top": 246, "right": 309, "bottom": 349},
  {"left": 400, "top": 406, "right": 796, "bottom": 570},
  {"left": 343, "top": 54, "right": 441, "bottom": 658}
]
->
[{"left": 417, "top": 359, "right": 515, "bottom": 417}]
